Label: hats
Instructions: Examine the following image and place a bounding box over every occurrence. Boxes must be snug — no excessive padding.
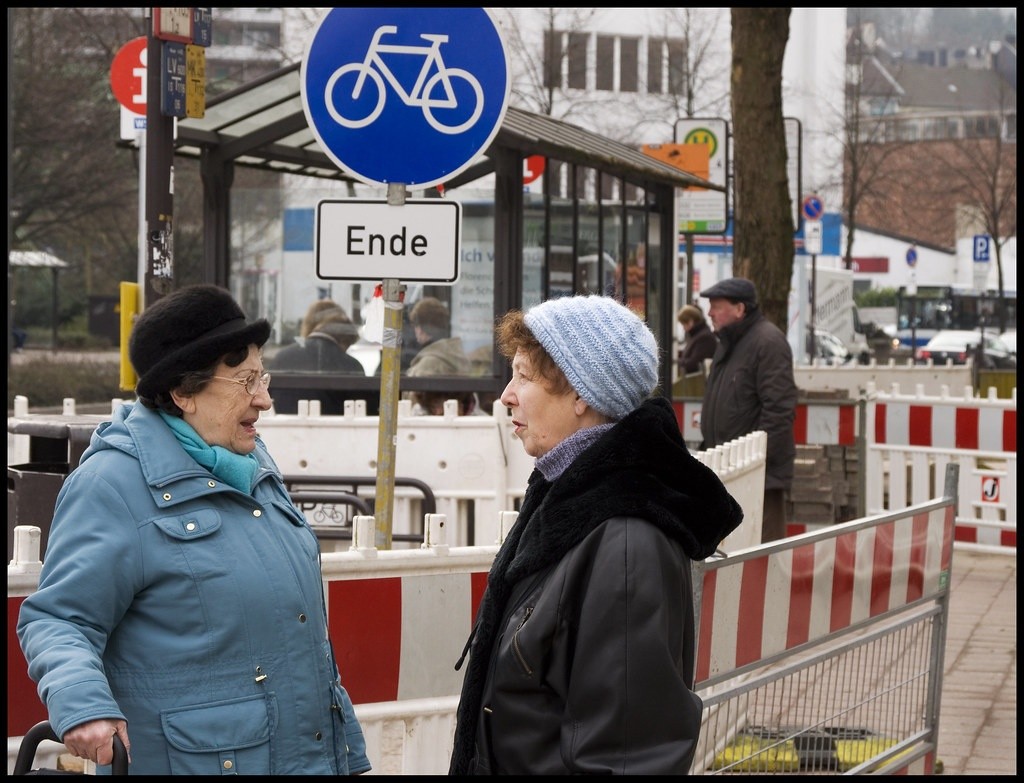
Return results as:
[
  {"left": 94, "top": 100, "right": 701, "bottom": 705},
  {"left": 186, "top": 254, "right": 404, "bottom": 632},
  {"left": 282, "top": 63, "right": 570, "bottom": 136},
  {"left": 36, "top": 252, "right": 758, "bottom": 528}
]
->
[
  {"left": 524, "top": 295, "right": 658, "bottom": 421},
  {"left": 129, "top": 282, "right": 272, "bottom": 397},
  {"left": 699, "top": 277, "right": 757, "bottom": 306}
]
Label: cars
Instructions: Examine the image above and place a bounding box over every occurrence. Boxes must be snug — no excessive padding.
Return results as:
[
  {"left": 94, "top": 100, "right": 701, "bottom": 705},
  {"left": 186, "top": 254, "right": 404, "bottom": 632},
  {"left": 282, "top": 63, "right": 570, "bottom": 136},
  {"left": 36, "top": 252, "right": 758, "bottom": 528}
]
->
[{"left": 915, "top": 329, "right": 1016, "bottom": 369}]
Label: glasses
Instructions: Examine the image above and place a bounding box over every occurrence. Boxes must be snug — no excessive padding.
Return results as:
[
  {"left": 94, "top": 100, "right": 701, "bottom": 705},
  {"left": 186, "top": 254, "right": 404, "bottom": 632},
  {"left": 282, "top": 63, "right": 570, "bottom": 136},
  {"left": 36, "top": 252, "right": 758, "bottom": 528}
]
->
[{"left": 213, "top": 372, "right": 271, "bottom": 395}]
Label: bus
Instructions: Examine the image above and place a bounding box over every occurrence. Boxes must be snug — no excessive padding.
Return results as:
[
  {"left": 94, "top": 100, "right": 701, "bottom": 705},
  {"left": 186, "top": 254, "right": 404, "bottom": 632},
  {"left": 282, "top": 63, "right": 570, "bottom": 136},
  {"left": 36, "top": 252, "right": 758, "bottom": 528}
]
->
[{"left": 892, "top": 284, "right": 1017, "bottom": 351}]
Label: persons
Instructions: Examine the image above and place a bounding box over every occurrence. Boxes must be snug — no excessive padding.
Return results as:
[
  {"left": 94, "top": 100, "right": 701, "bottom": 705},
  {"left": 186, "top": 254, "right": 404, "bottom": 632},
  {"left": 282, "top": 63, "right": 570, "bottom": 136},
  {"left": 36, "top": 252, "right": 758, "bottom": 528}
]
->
[
  {"left": 447, "top": 295, "right": 745, "bottom": 775},
  {"left": 270, "top": 300, "right": 368, "bottom": 415},
  {"left": 673, "top": 305, "right": 717, "bottom": 374},
  {"left": 14, "top": 284, "right": 373, "bottom": 775},
  {"left": 699, "top": 277, "right": 798, "bottom": 544},
  {"left": 402, "top": 299, "right": 487, "bottom": 416}
]
